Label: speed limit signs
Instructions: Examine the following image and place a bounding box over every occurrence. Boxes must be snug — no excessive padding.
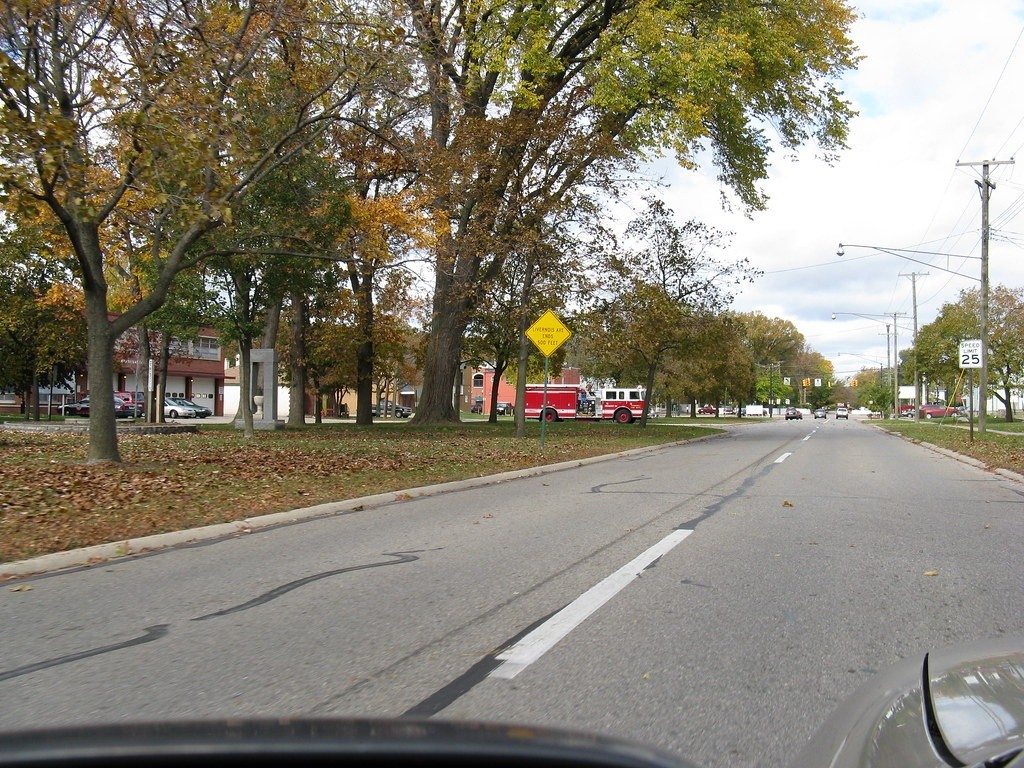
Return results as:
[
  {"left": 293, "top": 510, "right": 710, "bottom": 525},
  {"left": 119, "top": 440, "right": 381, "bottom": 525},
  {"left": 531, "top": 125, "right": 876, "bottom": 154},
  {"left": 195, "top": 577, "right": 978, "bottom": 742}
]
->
[{"left": 958, "top": 339, "right": 984, "bottom": 369}]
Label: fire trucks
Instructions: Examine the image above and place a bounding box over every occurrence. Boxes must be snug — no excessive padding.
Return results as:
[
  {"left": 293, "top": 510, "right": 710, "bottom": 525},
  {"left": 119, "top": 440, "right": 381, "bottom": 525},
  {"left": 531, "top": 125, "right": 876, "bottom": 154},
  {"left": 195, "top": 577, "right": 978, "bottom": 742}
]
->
[{"left": 525, "top": 379, "right": 656, "bottom": 424}]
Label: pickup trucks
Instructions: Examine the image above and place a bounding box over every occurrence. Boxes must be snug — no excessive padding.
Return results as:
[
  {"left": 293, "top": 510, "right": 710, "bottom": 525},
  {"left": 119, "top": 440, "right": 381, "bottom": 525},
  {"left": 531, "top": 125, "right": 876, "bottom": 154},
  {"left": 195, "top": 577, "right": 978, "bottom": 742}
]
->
[{"left": 371, "top": 398, "right": 413, "bottom": 418}]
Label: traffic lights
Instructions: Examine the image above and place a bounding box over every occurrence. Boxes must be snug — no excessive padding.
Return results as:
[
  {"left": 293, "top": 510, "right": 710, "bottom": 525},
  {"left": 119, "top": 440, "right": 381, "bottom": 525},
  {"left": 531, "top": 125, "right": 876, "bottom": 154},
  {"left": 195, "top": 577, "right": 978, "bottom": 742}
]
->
[
  {"left": 854, "top": 380, "right": 858, "bottom": 387},
  {"left": 850, "top": 380, "right": 854, "bottom": 387},
  {"left": 806, "top": 379, "right": 811, "bottom": 386},
  {"left": 802, "top": 379, "right": 806, "bottom": 387}
]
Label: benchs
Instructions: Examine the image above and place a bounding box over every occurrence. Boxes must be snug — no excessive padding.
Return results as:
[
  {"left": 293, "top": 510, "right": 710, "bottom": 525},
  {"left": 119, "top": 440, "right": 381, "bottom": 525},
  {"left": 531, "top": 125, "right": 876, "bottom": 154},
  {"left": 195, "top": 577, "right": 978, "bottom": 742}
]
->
[{"left": 338, "top": 403, "right": 347, "bottom": 418}]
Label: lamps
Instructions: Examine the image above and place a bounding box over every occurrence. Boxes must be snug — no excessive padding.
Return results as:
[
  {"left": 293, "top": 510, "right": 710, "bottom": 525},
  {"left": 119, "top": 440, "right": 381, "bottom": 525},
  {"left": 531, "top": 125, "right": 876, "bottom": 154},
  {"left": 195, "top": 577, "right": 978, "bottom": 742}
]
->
[
  {"left": 191, "top": 377, "right": 193, "bottom": 382},
  {"left": 80, "top": 374, "right": 83, "bottom": 377},
  {"left": 124, "top": 374, "right": 127, "bottom": 379}
]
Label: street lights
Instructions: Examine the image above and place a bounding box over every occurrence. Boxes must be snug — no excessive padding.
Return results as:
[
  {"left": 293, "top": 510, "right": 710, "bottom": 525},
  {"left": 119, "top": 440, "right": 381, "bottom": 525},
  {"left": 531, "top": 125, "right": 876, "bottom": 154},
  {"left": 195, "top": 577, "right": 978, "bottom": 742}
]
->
[
  {"left": 831, "top": 312, "right": 920, "bottom": 424},
  {"left": 836, "top": 240, "right": 991, "bottom": 435},
  {"left": 838, "top": 352, "right": 893, "bottom": 414}
]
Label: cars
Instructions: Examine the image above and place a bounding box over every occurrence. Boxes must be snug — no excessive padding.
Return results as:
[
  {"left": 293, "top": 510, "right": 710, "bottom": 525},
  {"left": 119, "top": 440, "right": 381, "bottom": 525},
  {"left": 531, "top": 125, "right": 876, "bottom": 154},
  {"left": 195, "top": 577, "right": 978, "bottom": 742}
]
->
[
  {"left": 813, "top": 409, "right": 828, "bottom": 419},
  {"left": 496, "top": 402, "right": 515, "bottom": 415},
  {"left": 835, "top": 407, "right": 849, "bottom": 419},
  {"left": 113, "top": 389, "right": 213, "bottom": 420},
  {"left": 470, "top": 405, "right": 482, "bottom": 413},
  {"left": 785, "top": 408, "right": 802, "bottom": 421},
  {"left": 902, "top": 409, "right": 915, "bottom": 418},
  {"left": 697, "top": 403, "right": 719, "bottom": 415},
  {"left": 919, "top": 403, "right": 959, "bottom": 419},
  {"left": 57, "top": 394, "right": 127, "bottom": 418},
  {"left": 723, "top": 405, "right": 768, "bottom": 416}
]
